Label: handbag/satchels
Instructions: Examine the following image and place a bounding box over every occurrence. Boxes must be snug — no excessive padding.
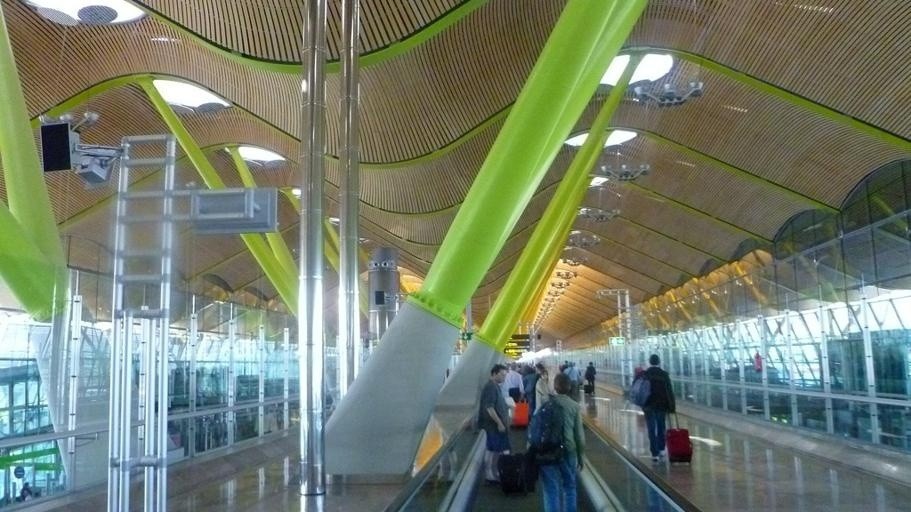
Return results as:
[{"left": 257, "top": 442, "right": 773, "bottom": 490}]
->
[{"left": 530, "top": 440, "right": 566, "bottom": 466}]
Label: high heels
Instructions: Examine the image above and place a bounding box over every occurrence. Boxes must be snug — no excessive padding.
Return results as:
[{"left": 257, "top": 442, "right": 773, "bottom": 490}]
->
[{"left": 484, "top": 477, "right": 499, "bottom": 485}]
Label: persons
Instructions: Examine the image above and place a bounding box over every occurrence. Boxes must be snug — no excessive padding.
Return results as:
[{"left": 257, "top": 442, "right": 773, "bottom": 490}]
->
[
  {"left": 478, "top": 364, "right": 516, "bottom": 486},
  {"left": 558, "top": 360, "right": 596, "bottom": 403},
  {"left": 529, "top": 372, "right": 586, "bottom": 512},
  {"left": 631, "top": 353, "right": 676, "bottom": 462},
  {"left": 500, "top": 360, "right": 551, "bottom": 420}
]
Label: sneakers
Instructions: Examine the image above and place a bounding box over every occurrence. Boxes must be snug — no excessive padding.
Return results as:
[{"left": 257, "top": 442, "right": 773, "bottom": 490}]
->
[
  {"left": 659, "top": 449, "right": 666, "bottom": 457},
  {"left": 651, "top": 454, "right": 660, "bottom": 462}
]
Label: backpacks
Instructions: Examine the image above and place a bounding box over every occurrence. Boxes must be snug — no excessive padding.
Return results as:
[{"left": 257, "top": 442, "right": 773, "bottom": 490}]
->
[{"left": 629, "top": 376, "right": 651, "bottom": 406}]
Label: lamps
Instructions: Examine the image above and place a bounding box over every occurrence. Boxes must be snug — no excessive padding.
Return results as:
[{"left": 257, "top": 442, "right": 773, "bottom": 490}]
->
[
  {"left": 592, "top": 94, "right": 657, "bottom": 184},
  {"left": 23, "top": 10, "right": 145, "bottom": 136},
  {"left": 600, "top": 1, "right": 709, "bottom": 112},
  {"left": 529, "top": 176, "right": 626, "bottom": 338}
]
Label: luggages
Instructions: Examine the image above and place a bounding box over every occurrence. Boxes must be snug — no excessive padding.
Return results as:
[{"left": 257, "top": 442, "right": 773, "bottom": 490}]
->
[
  {"left": 665, "top": 427, "right": 693, "bottom": 463},
  {"left": 583, "top": 384, "right": 594, "bottom": 393},
  {"left": 497, "top": 451, "right": 536, "bottom": 497},
  {"left": 511, "top": 399, "right": 530, "bottom": 428}
]
